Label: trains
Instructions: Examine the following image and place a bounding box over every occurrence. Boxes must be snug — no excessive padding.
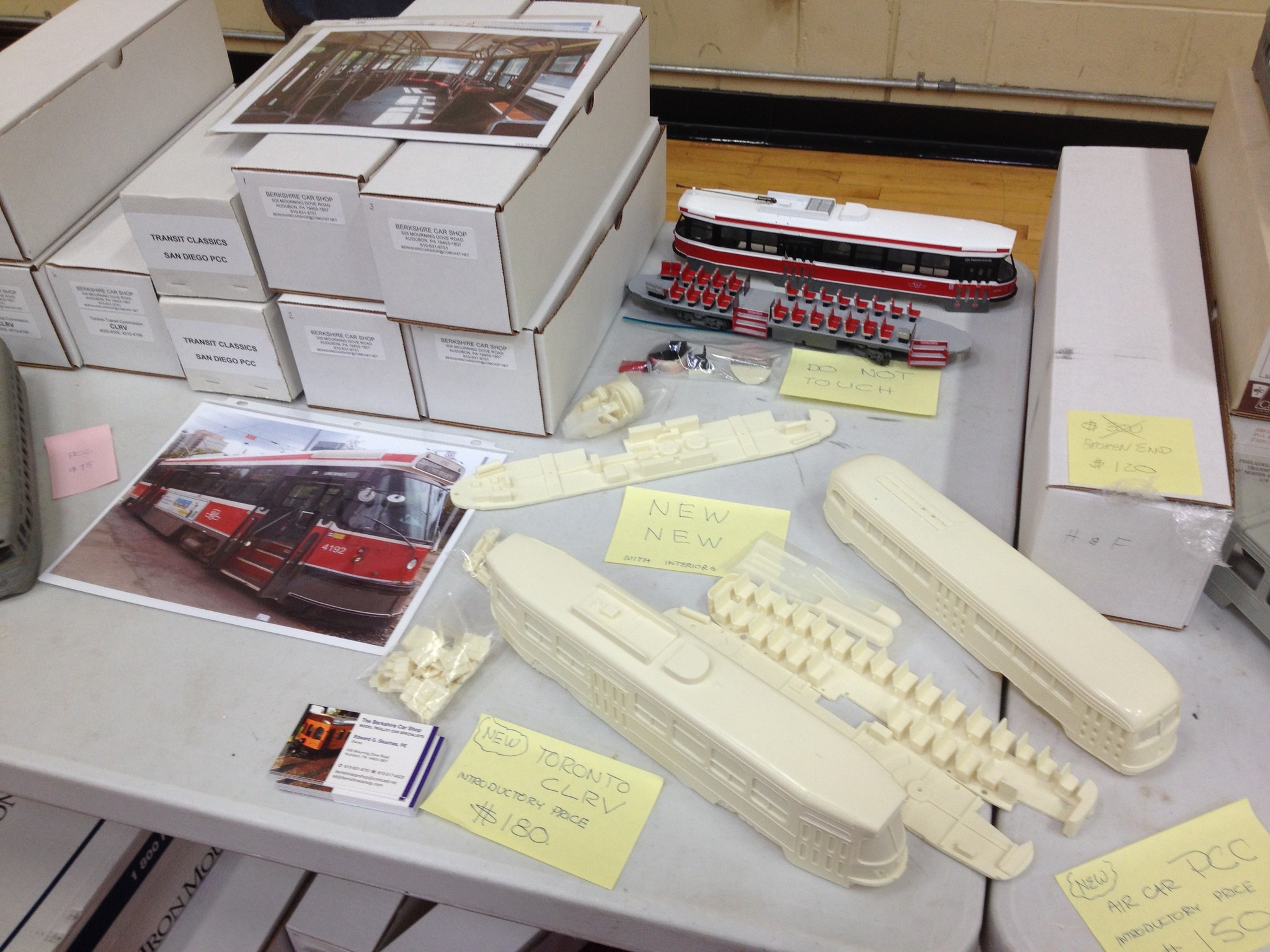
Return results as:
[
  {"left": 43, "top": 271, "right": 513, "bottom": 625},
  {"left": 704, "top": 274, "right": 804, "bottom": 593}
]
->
[{"left": 672, "top": 183, "right": 1018, "bottom": 309}]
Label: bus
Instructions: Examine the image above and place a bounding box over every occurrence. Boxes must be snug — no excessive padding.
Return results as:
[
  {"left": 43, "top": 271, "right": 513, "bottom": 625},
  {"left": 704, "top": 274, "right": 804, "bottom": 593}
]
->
[
  {"left": 293, "top": 703, "right": 358, "bottom": 758},
  {"left": 122, "top": 449, "right": 467, "bottom": 629}
]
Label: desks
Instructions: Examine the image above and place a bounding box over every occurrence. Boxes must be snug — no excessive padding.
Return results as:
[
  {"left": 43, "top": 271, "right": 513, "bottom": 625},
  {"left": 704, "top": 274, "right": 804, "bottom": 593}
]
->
[
  {"left": 0, "top": 225, "right": 1034, "bottom": 952},
  {"left": 986, "top": 591, "right": 1270, "bottom": 952}
]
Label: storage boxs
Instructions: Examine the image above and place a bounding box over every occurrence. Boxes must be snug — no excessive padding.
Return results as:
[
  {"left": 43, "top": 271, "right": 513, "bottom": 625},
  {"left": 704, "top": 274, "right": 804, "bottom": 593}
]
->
[
  {"left": 286, "top": 873, "right": 430, "bottom": 952},
  {"left": 122, "top": 19, "right": 350, "bottom": 307},
  {"left": 228, "top": 2, "right": 528, "bottom": 303},
  {"left": 0, "top": 0, "right": 230, "bottom": 262},
  {"left": 273, "top": 295, "right": 428, "bottom": 424},
  {"left": 1018, "top": 143, "right": 1232, "bottom": 627},
  {"left": 158, "top": 297, "right": 306, "bottom": 403},
  {"left": 362, "top": 1, "right": 650, "bottom": 323},
  {"left": 420, "top": 127, "right": 669, "bottom": 436},
  {"left": 151, "top": 849, "right": 314, "bottom": 952},
  {"left": 45, "top": 198, "right": 183, "bottom": 380},
  {"left": 0, "top": 808, "right": 220, "bottom": 952},
  {"left": 0, "top": 83, "right": 234, "bottom": 368},
  {"left": 377, "top": 903, "right": 589, "bottom": 952}
]
